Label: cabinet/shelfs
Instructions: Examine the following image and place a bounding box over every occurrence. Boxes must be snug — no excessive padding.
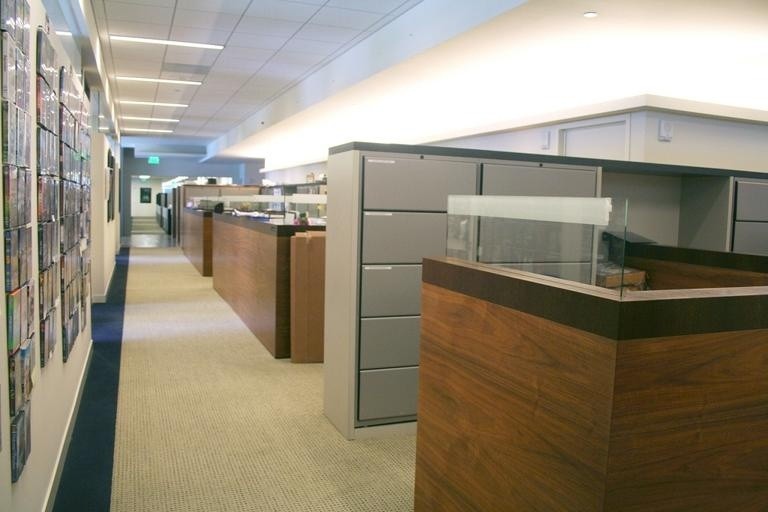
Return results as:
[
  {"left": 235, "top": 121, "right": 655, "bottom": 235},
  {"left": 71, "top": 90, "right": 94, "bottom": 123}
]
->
[
  {"left": 732, "top": 176, "right": 768, "bottom": 257},
  {"left": 171, "top": 184, "right": 260, "bottom": 247},
  {"left": 477, "top": 159, "right": 594, "bottom": 290},
  {"left": 357, "top": 144, "right": 477, "bottom": 443}
]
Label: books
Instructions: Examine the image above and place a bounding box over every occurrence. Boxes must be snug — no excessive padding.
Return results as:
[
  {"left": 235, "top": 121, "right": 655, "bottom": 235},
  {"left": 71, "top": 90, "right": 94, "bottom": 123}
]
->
[
  {"left": 596, "top": 260, "right": 648, "bottom": 292},
  {"left": 3, "top": 2, "right": 34, "bottom": 482},
  {"left": 36, "top": 25, "right": 89, "bottom": 367}
]
